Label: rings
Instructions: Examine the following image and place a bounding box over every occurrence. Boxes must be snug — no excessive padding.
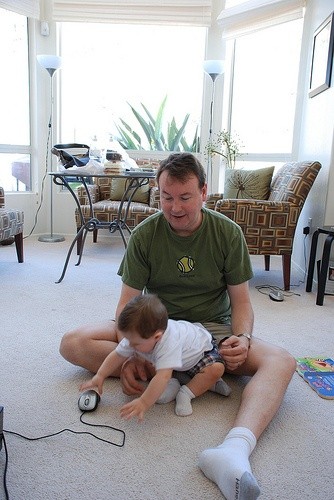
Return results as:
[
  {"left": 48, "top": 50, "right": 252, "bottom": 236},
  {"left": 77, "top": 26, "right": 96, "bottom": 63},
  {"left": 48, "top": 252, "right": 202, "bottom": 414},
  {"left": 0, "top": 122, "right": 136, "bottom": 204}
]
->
[{"left": 237, "top": 362, "right": 240, "bottom": 368}]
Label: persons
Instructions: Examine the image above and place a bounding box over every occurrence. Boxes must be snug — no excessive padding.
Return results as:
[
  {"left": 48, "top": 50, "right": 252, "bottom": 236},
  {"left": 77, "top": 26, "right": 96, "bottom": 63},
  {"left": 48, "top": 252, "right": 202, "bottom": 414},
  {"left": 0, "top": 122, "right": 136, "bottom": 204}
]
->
[
  {"left": 79, "top": 293, "right": 226, "bottom": 417},
  {"left": 59, "top": 153, "right": 295, "bottom": 500}
]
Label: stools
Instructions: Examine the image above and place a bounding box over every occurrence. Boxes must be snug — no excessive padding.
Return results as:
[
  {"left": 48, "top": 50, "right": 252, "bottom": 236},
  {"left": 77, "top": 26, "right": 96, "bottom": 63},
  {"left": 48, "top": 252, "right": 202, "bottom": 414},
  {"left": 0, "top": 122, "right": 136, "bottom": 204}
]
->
[{"left": 305, "top": 225, "right": 334, "bottom": 306}]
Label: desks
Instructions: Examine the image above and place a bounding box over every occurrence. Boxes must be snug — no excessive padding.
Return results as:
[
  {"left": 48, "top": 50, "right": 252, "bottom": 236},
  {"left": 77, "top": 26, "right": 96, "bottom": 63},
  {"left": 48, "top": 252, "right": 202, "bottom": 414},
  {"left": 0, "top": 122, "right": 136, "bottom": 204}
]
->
[{"left": 47, "top": 168, "right": 158, "bottom": 284}]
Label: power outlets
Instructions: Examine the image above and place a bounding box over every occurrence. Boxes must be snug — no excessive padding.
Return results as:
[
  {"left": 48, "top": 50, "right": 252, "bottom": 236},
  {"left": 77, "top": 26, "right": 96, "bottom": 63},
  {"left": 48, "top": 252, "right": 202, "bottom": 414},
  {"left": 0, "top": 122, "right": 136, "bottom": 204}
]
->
[{"left": 305, "top": 218, "right": 312, "bottom": 236}]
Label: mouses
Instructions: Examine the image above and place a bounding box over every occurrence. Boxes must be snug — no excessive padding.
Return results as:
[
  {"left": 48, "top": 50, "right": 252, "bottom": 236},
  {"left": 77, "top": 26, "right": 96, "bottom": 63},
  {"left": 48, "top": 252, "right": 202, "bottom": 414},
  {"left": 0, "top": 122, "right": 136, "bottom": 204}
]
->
[{"left": 78, "top": 388, "right": 100, "bottom": 412}]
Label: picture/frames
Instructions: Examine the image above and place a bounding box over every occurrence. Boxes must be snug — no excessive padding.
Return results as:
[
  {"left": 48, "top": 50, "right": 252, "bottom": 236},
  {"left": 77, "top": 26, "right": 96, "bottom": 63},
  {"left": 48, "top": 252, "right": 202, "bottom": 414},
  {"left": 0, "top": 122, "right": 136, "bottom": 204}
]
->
[{"left": 309, "top": 10, "right": 334, "bottom": 96}]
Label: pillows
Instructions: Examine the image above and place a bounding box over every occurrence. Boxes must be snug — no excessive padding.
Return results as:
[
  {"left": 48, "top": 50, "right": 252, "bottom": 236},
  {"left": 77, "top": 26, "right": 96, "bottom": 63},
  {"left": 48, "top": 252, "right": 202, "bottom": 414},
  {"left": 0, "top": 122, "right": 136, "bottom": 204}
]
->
[
  {"left": 109, "top": 170, "right": 150, "bottom": 204},
  {"left": 223, "top": 165, "right": 275, "bottom": 201}
]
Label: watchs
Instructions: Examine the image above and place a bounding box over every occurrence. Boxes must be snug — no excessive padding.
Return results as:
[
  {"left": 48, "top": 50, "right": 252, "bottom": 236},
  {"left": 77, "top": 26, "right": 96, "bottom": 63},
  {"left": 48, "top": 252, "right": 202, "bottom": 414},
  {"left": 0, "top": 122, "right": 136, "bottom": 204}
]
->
[{"left": 237, "top": 333, "right": 251, "bottom": 349}]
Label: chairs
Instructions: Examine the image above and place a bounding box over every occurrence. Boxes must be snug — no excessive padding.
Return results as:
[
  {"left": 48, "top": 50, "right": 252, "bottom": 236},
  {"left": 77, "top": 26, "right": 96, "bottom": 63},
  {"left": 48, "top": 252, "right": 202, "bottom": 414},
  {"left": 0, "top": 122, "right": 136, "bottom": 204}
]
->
[
  {"left": 206, "top": 159, "right": 322, "bottom": 291},
  {"left": 0, "top": 187, "right": 25, "bottom": 263},
  {"left": 12, "top": 162, "right": 30, "bottom": 192},
  {"left": 74, "top": 149, "right": 163, "bottom": 256}
]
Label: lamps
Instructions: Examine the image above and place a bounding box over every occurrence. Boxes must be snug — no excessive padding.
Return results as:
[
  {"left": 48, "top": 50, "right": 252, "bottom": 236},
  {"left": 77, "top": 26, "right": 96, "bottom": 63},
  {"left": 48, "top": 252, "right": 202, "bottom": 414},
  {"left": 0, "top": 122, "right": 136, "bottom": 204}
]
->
[
  {"left": 37, "top": 54, "right": 66, "bottom": 243},
  {"left": 203, "top": 60, "right": 225, "bottom": 194}
]
